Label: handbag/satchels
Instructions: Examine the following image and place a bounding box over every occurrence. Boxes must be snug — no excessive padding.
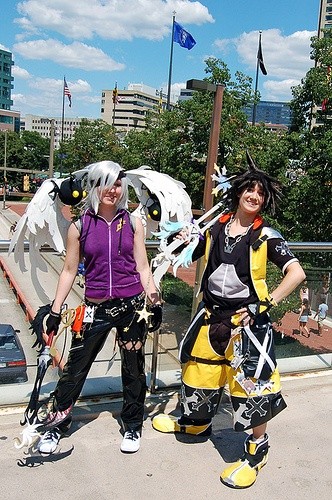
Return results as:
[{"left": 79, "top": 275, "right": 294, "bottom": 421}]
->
[{"left": 308, "top": 305, "right": 312, "bottom": 316}]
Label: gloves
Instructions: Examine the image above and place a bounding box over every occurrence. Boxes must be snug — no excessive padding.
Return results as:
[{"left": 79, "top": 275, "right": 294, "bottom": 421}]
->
[
  {"left": 45, "top": 309, "right": 62, "bottom": 336},
  {"left": 148, "top": 304, "right": 163, "bottom": 333}
]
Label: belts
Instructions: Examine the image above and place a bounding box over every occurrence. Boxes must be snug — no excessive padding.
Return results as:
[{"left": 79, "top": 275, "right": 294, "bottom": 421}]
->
[{"left": 94, "top": 291, "right": 145, "bottom": 319}]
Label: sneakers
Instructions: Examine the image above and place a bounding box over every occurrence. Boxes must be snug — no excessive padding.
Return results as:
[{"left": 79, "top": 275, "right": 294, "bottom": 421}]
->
[
  {"left": 120, "top": 429, "right": 142, "bottom": 453},
  {"left": 39, "top": 429, "right": 63, "bottom": 454}
]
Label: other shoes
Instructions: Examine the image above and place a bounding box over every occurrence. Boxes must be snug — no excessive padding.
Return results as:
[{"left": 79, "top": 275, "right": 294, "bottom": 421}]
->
[
  {"left": 152, "top": 413, "right": 213, "bottom": 436},
  {"left": 307, "top": 334, "right": 310, "bottom": 338},
  {"left": 220, "top": 433, "right": 271, "bottom": 489},
  {"left": 298, "top": 334, "right": 302, "bottom": 337}
]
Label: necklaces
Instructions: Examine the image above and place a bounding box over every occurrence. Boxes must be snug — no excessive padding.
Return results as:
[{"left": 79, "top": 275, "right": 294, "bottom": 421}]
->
[{"left": 224, "top": 219, "right": 255, "bottom": 254}]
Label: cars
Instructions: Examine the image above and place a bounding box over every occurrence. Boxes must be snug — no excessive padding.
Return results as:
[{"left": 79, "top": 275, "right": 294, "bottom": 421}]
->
[{"left": 0, "top": 323, "right": 28, "bottom": 384}]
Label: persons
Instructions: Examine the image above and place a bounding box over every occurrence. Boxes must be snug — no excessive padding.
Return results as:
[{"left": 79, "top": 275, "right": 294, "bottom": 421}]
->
[
  {"left": 7, "top": 160, "right": 193, "bottom": 453},
  {"left": 152, "top": 171, "right": 306, "bottom": 489},
  {"left": 297, "top": 283, "right": 328, "bottom": 338}
]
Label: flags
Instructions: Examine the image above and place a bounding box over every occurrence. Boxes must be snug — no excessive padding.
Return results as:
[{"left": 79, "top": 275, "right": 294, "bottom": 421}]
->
[
  {"left": 112, "top": 82, "right": 118, "bottom": 103},
  {"left": 158, "top": 90, "right": 163, "bottom": 109},
  {"left": 257, "top": 34, "right": 267, "bottom": 75},
  {"left": 172, "top": 21, "right": 196, "bottom": 50},
  {"left": 64, "top": 78, "right": 72, "bottom": 108}
]
